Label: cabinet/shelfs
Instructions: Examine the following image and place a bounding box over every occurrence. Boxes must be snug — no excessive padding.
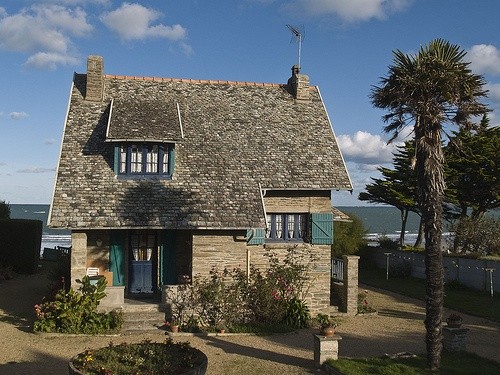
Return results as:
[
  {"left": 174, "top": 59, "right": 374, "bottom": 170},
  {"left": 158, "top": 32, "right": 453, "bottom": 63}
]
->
[
  {"left": 312, "top": 333, "right": 342, "bottom": 369},
  {"left": 441, "top": 325, "right": 470, "bottom": 353}
]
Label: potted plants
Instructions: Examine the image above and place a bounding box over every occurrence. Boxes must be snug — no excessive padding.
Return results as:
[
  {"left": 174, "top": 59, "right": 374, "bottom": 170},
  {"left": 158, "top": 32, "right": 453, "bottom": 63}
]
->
[
  {"left": 67, "top": 336, "right": 209, "bottom": 375},
  {"left": 218, "top": 324, "right": 225, "bottom": 333},
  {"left": 315, "top": 314, "right": 338, "bottom": 338},
  {"left": 447, "top": 313, "right": 463, "bottom": 327},
  {"left": 169, "top": 319, "right": 179, "bottom": 332}
]
